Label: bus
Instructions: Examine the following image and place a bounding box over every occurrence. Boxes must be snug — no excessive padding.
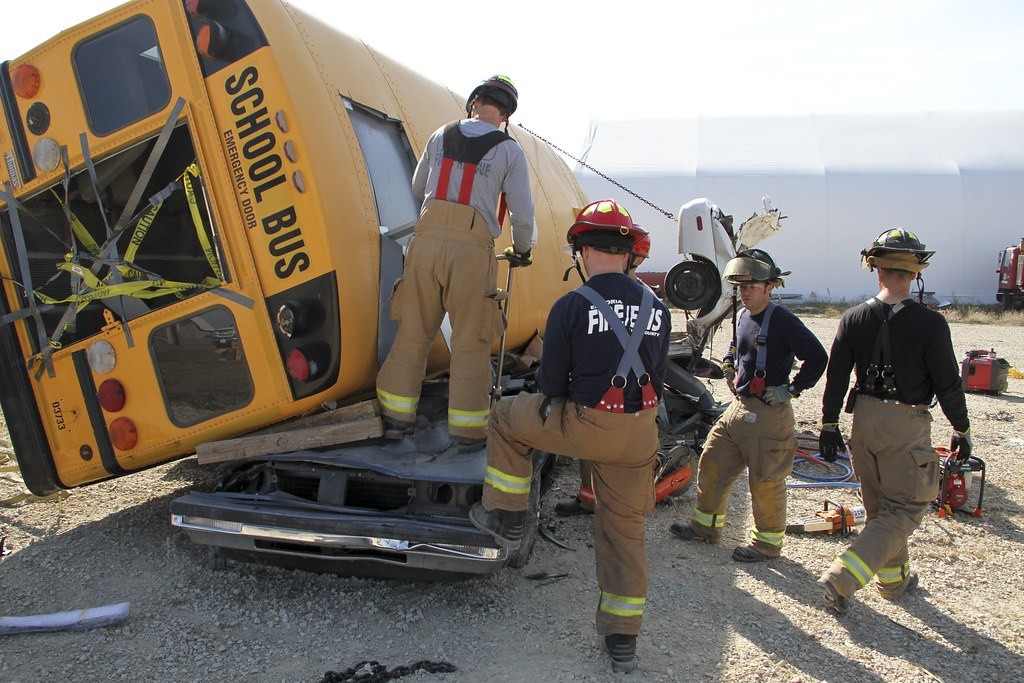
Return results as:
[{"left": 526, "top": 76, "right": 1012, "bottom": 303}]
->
[{"left": 0, "top": 0, "right": 600, "bottom": 496}]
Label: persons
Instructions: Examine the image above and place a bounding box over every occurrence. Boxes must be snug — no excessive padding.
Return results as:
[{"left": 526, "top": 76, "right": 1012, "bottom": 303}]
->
[
  {"left": 818, "top": 227, "right": 973, "bottom": 613},
  {"left": 668, "top": 249, "right": 828, "bottom": 563},
  {"left": 468, "top": 199, "right": 672, "bottom": 674},
  {"left": 554, "top": 223, "right": 656, "bottom": 517},
  {"left": 376, "top": 75, "right": 535, "bottom": 454}
]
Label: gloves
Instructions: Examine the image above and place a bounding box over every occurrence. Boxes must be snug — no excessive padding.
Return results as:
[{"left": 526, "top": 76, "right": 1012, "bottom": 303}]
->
[
  {"left": 950, "top": 426, "right": 972, "bottom": 465},
  {"left": 763, "top": 383, "right": 795, "bottom": 406},
  {"left": 818, "top": 422, "right": 846, "bottom": 462},
  {"left": 503, "top": 243, "right": 532, "bottom": 268},
  {"left": 721, "top": 362, "right": 737, "bottom": 396}
]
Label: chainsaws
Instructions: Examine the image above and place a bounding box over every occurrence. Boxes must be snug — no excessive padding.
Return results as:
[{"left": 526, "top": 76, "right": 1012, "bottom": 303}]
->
[{"left": 654, "top": 440, "right": 697, "bottom": 505}]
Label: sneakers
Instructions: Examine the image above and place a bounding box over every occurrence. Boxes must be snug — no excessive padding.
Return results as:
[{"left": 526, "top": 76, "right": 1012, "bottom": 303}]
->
[
  {"left": 458, "top": 436, "right": 488, "bottom": 452},
  {"left": 904, "top": 572, "right": 919, "bottom": 591},
  {"left": 732, "top": 546, "right": 768, "bottom": 561},
  {"left": 669, "top": 521, "right": 711, "bottom": 543},
  {"left": 556, "top": 500, "right": 594, "bottom": 515},
  {"left": 602, "top": 632, "right": 636, "bottom": 672},
  {"left": 384, "top": 419, "right": 414, "bottom": 440},
  {"left": 816, "top": 578, "right": 849, "bottom": 612},
  {"left": 469, "top": 500, "right": 524, "bottom": 550}
]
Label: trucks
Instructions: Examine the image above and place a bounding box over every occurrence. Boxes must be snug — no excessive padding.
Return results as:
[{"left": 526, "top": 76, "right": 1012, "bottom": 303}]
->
[{"left": 994, "top": 238, "right": 1024, "bottom": 308}]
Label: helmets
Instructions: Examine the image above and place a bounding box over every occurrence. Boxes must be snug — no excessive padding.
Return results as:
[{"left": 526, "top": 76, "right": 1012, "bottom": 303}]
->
[
  {"left": 630, "top": 224, "right": 650, "bottom": 258},
  {"left": 567, "top": 198, "right": 649, "bottom": 252},
  {"left": 466, "top": 75, "right": 518, "bottom": 118},
  {"left": 721, "top": 249, "right": 781, "bottom": 284},
  {"left": 865, "top": 227, "right": 936, "bottom": 261}
]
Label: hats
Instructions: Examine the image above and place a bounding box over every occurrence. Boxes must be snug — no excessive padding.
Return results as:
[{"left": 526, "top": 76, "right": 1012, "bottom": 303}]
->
[{"left": 867, "top": 253, "right": 929, "bottom": 273}]
